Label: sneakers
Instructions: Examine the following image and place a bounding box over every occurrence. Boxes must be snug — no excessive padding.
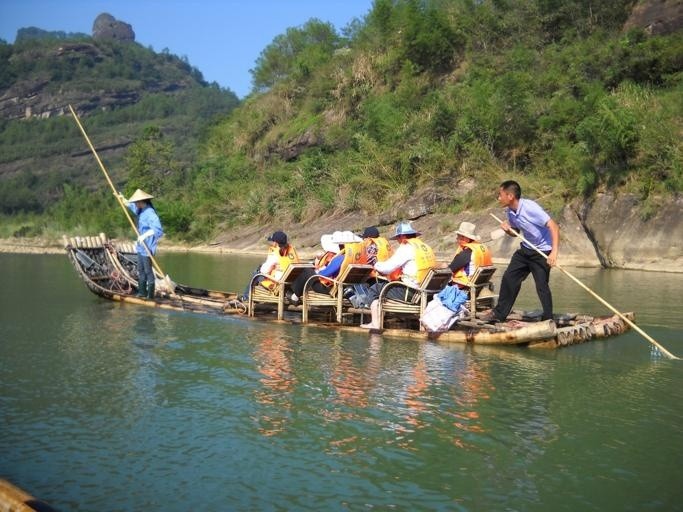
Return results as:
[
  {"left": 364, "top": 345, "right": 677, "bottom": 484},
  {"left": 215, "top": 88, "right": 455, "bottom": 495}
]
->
[
  {"left": 542, "top": 315, "right": 553, "bottom": 320},
  {"left": 480, "top": 311, "right": 502, "bottom": 322}
]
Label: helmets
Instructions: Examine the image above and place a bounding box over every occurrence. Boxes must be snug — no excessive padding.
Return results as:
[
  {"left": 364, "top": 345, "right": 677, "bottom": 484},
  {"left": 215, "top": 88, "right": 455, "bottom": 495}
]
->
[
  {"left": 453, "top": 222, "right": 481, "bottom": 241},
  {"left": 390, "top": 223, "right": 421, "bottom": 239},
  {"left": 359, "top": 227, "right": 380, "bottom": 238}
]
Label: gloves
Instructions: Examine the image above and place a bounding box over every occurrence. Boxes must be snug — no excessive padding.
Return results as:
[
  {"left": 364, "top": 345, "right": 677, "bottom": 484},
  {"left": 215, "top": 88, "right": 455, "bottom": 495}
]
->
[
  {"left": 117, "top": 191, "right": 129, "bottom": 207},
  {"left": 138, "top": 229, "right": 153, "bottom": 245}
]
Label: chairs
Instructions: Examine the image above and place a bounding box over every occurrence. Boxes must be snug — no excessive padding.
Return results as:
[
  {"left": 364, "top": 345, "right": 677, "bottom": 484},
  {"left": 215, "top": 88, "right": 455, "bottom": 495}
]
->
[
  {"left": 248, "top": 263, "right": 316, "bottom": 320},
  {"left": 377, "top": 268, "right": 452, "bottom": 331},
  {"left": 303, "top": 264, "right": 373, "bottom": 323},
  {"left": 449, "top": 266, "right": 497, "bottom": 321}
]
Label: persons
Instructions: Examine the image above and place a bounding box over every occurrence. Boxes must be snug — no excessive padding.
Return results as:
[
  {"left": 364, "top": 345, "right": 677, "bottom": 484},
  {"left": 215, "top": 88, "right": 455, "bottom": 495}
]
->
[
  {"left": 314, "top": 236, "right": 338, "bottom": 269},
  {"left": 477, "top": 181, "right": 560, "bottom": 323},
  {"left": 238, "top": 232, "right": 299, "bottom": 302},
  {"left": 359, "top": 223, "right": 435, "bottom": 329},
  {"left": 361, "top": 227, "right": 393, "bottom": 278},
  {"left": 113, "top": 189, "right": 163, "bottom": 298},
  {"left": 451, "top": 222, "right": 491, "bottom": 290},
  {"left": 286, "top": 233, "right": 367, "bottom": 306}
]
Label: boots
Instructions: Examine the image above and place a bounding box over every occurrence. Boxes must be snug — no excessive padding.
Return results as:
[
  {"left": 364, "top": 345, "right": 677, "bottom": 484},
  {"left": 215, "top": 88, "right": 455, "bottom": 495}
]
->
[
  {"left": 145, "top": 282, "right": 155, "bottom": 300},
  {"left": 361, "top": 299, "right": 384, "bottom": 330},
  {"left": 136, "top": 282, "right": 147, "bottom": 297}
]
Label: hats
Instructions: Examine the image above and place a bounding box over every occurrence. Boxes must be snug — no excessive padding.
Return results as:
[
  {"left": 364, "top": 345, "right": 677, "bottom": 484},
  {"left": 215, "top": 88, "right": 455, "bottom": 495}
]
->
[
  {"left": 128, "top": 189, "right": 154, "bottom": 202},
  {"left": 268, "top": 231, "right": 287, "bottom": 243},
  {"left": 322, "top": 230, "right": 362, "bottom": 254}
]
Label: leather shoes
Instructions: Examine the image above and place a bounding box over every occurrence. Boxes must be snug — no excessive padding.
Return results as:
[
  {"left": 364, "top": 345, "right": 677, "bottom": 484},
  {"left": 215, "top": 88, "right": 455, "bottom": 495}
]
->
[{"left": 287, "top": 289, "right": 301, "bottom": 306}]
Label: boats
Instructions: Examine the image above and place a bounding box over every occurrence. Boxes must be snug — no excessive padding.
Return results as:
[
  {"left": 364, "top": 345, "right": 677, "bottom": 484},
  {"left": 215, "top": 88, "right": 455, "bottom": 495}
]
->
[{"left": 61, "top": 233, "right": 634, "bottom": 348}]
